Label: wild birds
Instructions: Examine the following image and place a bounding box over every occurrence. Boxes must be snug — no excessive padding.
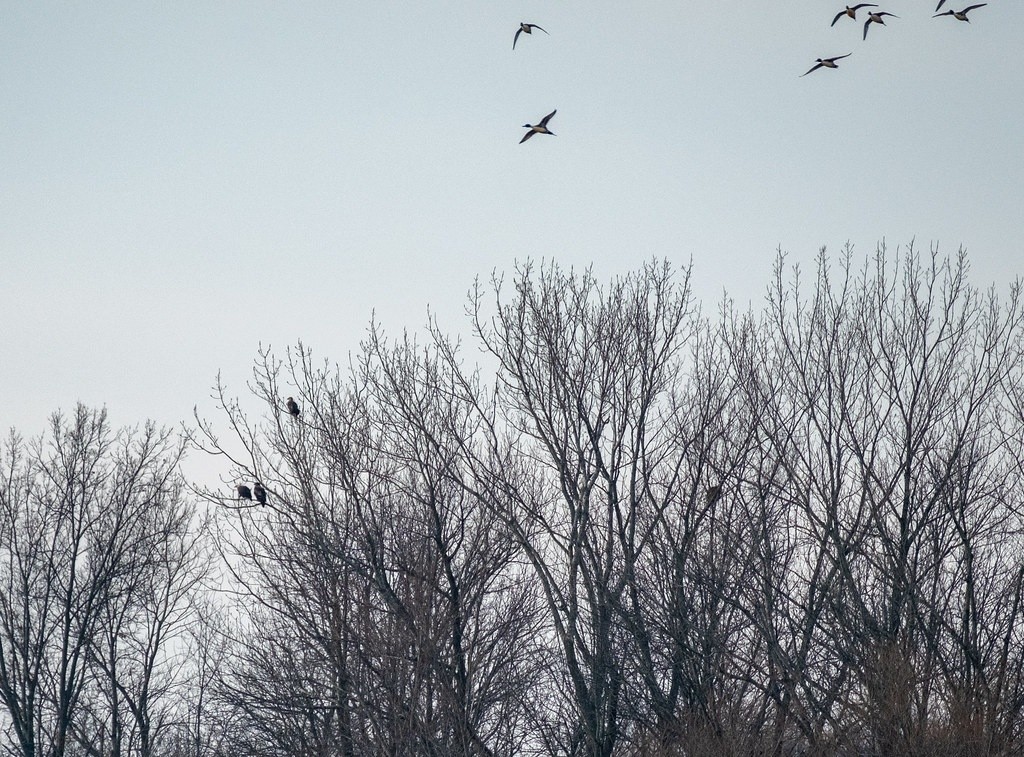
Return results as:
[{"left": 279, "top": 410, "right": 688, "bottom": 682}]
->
[
  {"left": 830, "top": 3, "right": 902, "bottom": 41},
  {"left": 798, "top": 52, "right": 852, "bottom": 76},
  {"left": 705, "top": 485, "right": 723, "bottom": 504},
  {"left": 518, "top": 108, "right": 558, "bottom": 146},
  {"left": 237, "top": 482, "right": 268, "bottom": 508},
  {"left": 286, "top": 396, "right": 301, "bottom": 424},
  {"left": 512, "top": 22, "right": 551, "bottom": 51},
  {"left": 931, "top": 0, "right": 988, "bottom": 25}
]
